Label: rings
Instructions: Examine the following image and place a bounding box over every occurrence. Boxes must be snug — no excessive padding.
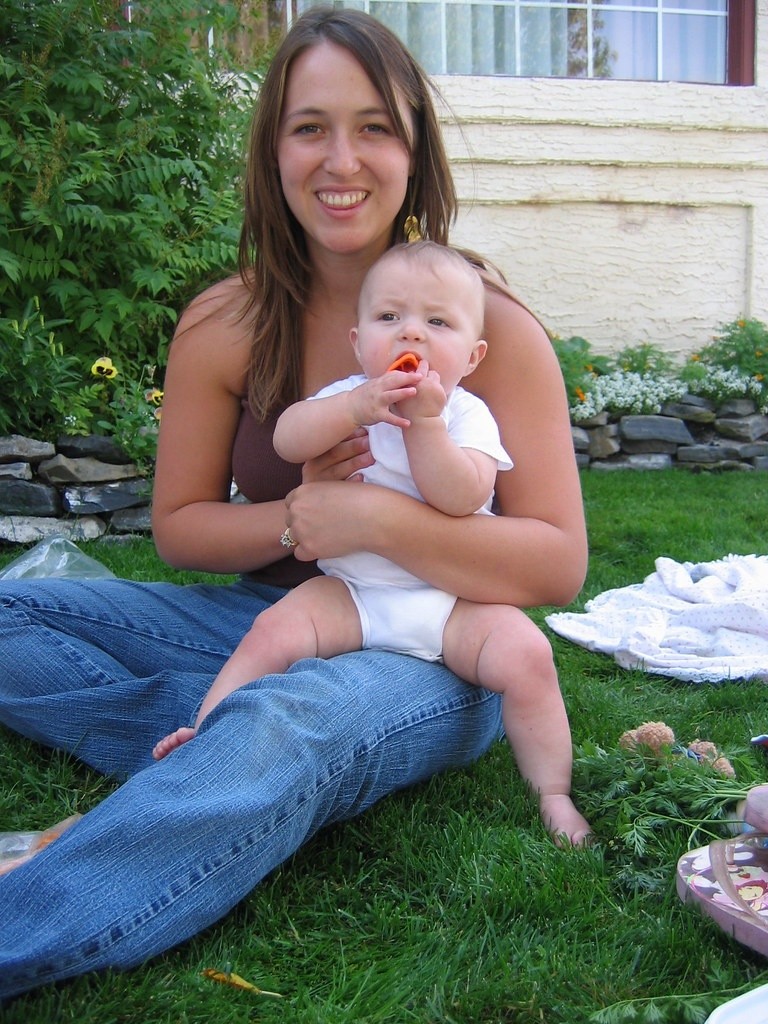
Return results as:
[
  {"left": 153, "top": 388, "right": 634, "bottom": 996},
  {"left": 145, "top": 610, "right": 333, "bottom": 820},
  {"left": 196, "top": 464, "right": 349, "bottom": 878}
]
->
[{"left": 280, "top": 527, "right": 300, "bottom": 549}]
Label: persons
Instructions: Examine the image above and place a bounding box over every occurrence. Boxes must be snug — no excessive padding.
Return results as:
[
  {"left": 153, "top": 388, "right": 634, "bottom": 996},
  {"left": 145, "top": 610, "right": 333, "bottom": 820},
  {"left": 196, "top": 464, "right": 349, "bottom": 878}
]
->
[
  {"left": 150, "top": 240, "right": 594, "bottom": 847},
  {"left": 0, "top": 15, "right": 587, "bottom": 991}
]
